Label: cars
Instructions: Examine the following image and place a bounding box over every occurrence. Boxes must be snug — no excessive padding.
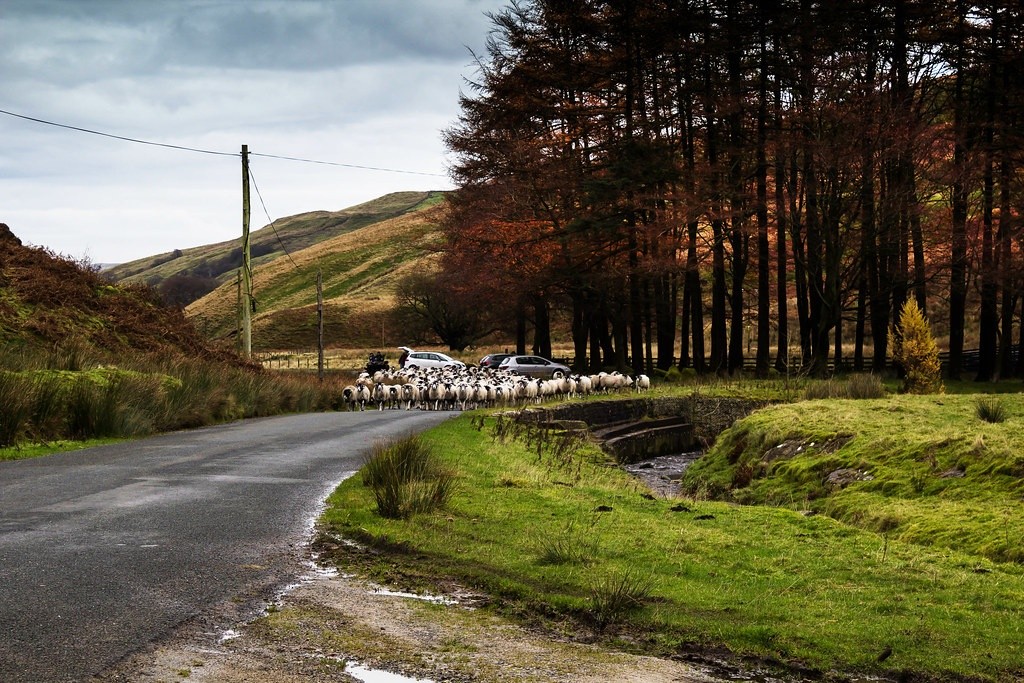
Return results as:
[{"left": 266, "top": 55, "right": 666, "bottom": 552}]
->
[
  {"left": 398, "top": 347, "right": 467, "bottom": 371},
  {"left": 499, "top": 355, "right": 572, "bottom": 380},
  {"left": 479, "top": 353, "right": 516, "bottom": 370}
]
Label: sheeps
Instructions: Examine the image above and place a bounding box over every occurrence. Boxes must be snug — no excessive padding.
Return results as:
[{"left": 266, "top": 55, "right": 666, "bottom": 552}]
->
[{"left": 342, "top": 364, "right": 650, "bottom": 413}]
